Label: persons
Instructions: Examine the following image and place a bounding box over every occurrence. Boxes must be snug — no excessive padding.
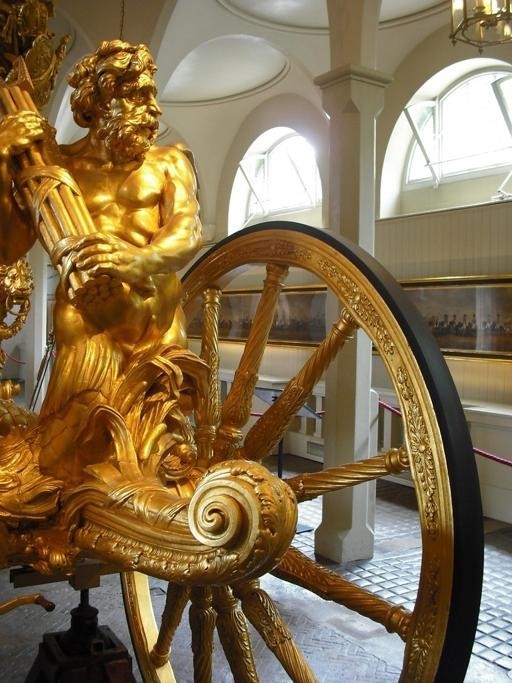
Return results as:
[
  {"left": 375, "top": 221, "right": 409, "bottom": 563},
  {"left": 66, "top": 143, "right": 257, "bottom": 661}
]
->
[
  {"left": 424, "top": 312, "right": 507, "bottom": 337},
  {"left": 0, "top": 40, "right": 206, "bottom": 406}
]
